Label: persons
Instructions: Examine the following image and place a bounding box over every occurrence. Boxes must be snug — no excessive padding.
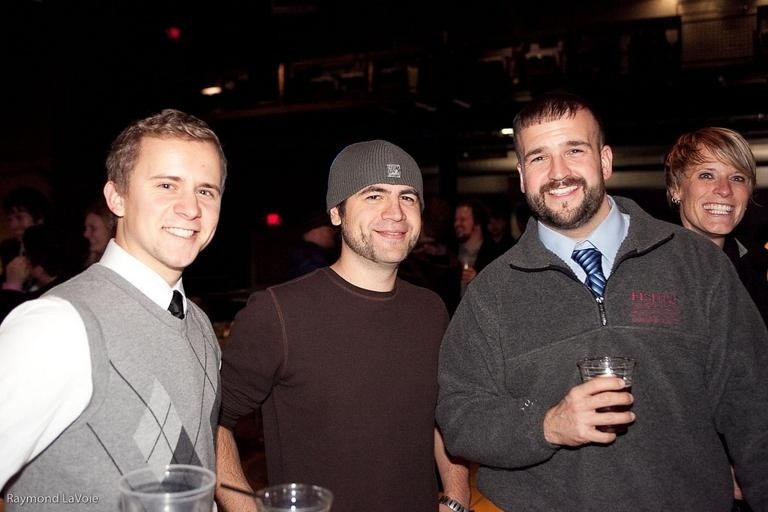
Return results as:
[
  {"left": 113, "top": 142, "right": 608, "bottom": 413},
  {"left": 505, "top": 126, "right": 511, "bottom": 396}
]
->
[
  {"left": 434, "top": 93, "right": 766, "bottom": 511},
  {"left": 82, "top": 201, "right": 118, "bottom": 268},
  {"left": 211, "top": 139, "right": 472, "bottom": 512},
  {"left": 667, "top": 128, "right": 767, "bottom": 326},
  {"left": 0, "top": 222, "right": 92, "bottom": 325},
  {"left": 1, "top": 107, "right": 226, "bottom": 512},
  {"left": 1, "top": 187, "right": 50, "bottom": 282},
  {"left": 400, "top": 201, "right": 532, "bottom": 314}
]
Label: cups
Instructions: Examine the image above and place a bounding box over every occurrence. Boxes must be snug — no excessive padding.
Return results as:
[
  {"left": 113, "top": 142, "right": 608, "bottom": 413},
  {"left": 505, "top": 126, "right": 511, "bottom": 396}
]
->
[
  {"left": 116, "top": 465, "right": 216, "bottom": 512},
  {"left": 576, "top": 355, "right": 635, "bottom": 435},
  {"left": 254, "top": 485, "right": 334, "bottom": 512}
]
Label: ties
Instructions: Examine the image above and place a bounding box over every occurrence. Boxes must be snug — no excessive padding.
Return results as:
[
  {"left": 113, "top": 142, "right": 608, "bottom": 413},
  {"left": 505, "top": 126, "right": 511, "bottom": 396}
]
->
[
  {"left": 163, "top": 290, "right": 183, "bottom": 323},
  {"left": 573, "top": 245, "right": 609, "bottom": 306}
]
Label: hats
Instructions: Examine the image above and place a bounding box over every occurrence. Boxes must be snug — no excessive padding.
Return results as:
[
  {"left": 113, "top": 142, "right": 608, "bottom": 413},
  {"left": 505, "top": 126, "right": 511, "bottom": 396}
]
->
[{"left": 324, "top": 140, "right": 425, "bottom": 212}]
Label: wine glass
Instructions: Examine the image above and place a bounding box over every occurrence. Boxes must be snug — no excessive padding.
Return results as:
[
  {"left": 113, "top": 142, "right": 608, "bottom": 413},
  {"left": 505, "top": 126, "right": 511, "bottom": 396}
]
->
[{"left": 461, "top": 259, "right": 472, "bottom": 288}]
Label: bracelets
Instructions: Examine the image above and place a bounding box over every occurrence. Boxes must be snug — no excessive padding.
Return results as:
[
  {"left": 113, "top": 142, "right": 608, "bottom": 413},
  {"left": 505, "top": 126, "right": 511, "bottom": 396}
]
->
[{"left": 432, "top": 493, "right": 467, "bottom": 512}]
